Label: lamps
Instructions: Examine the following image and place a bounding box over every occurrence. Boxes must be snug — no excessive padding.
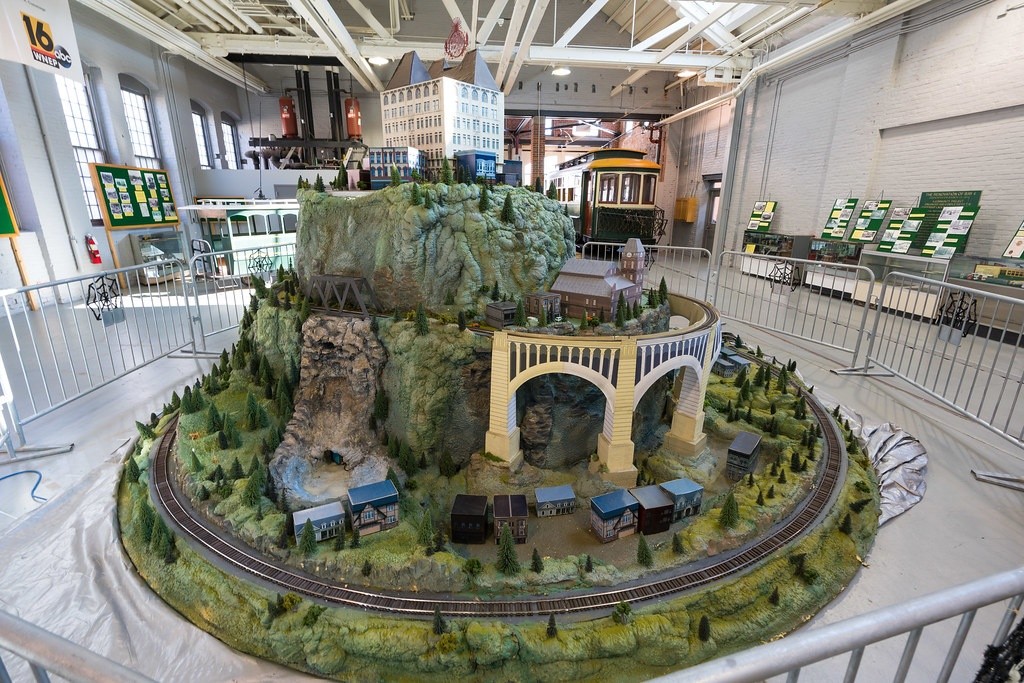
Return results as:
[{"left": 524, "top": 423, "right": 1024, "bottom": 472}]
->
[
  {"left": 367, "top": 56, "right": 390, "bottom": 66},
  {"left": 551, "top": 66, "right": 571, "bottom": 77},
  {"left": 674, "top": 23, "right": 698, "bottom": 78}
]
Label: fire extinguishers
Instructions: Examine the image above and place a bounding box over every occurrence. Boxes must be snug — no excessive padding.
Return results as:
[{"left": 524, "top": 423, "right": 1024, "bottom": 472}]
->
[{"left": 85, "top": 234, "right": 102, "bottom": 264}]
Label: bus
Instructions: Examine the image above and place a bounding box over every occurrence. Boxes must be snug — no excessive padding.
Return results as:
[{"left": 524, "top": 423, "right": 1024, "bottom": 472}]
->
[{"left": 544, "top": 148, "right": 662, "bottom": 258}]
